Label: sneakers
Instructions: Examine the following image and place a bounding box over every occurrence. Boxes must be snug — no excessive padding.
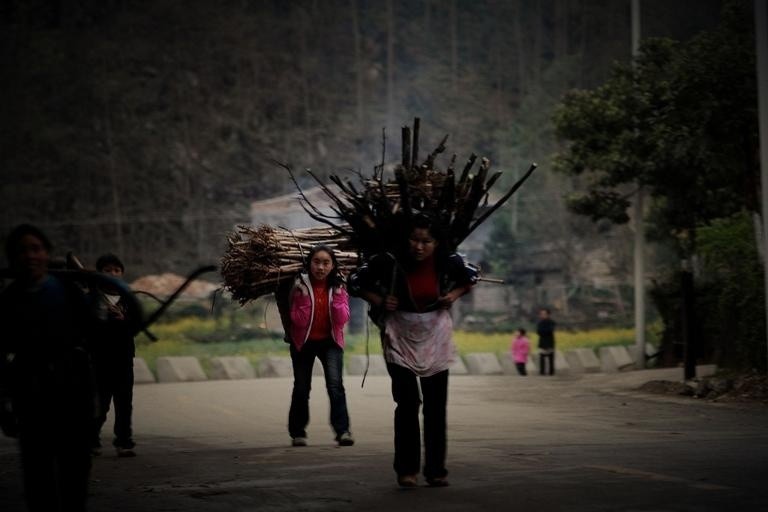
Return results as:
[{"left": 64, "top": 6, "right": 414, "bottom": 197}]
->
[
  {"left": 116, "top": 446, "right": 136, "bottom": 455},
  {"left": 292, "top": 436, "right": 307, "bottom": 445},
  {"left": 338, "top": 433, "right": 354, "bottom": 445},
  {"left": 398, "top": 474, "right": 447, "bottom": 488}
]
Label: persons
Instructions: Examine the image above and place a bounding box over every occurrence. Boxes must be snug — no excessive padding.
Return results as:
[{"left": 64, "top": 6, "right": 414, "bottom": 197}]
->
[
  {"left": 0, "top": 223, "right": 124, "bottom": 512},
  {"left": 78, "top": 253, "right": 144, "bottom": 458},
  {"left": 346, "top": 219, "right": 480, "bottom": 487},
  {"left": 512, "top": 327, "right": 530, "bottom": 375},
  {"left": 271, "top": 245, "right": 355, "bottom": 446},
  {"left": 536, "top": 307, "right": 556, "bottom": 375}
]
